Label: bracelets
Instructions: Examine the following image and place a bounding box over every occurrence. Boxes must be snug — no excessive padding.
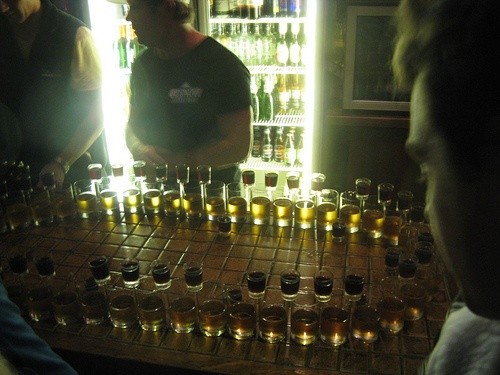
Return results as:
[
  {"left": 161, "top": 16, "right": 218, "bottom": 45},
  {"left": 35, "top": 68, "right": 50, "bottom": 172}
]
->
[{"left": 56, "top": 157, "right": 71, "bottom": 175}]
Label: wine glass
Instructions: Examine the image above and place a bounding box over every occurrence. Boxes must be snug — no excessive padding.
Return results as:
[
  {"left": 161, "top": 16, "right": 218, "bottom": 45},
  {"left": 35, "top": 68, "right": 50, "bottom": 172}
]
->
[{"left": 354, "top": 177, "right": 413, "bottom": 219}]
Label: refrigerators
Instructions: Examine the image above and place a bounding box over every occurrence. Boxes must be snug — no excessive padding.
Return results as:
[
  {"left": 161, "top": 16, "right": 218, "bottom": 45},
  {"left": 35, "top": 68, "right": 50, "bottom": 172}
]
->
[{"left": 104, "top": 0, "right": 316, "bottom": 194}]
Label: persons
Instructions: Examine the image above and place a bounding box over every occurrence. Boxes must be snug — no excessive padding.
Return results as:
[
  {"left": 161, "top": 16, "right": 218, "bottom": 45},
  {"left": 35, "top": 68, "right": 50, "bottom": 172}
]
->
[
  {"left": 393, "top": 0, "right": 500, "bottom": 375},
  {"left": 0, "top": 0, "right": 104, "bottom": 195},
  {"left": 109, "top": 0, "right": 254, "bottom": 197},
  {"left": 0, "top": 99, "right": 77, "bottom": 375}
]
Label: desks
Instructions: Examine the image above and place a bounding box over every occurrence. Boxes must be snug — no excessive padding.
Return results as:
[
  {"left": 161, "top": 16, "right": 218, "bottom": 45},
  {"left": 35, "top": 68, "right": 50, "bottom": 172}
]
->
[
  {"left": 324, "top": 110, "right": 426, "bottom": 201},
  {"left": 0, "top": 204, "right": 460, "bottom": 375}
]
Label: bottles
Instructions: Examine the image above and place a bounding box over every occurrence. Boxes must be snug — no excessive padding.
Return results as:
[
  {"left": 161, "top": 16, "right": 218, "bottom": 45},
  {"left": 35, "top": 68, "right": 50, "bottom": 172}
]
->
[
  {"left": 252, "top": 125, "right": 305, "bottom": 166},
  {"left": 211, "top": 23, "right": 305, "bottom": 67},
  {"left": 250, "top": 74, "right": 304, "bottom": 120},
  {"left": 117, "top": 23, "right": 141, "bottom": 68},
  {"left": 210, "top": 0, "right": 307, "bottom": 18}
]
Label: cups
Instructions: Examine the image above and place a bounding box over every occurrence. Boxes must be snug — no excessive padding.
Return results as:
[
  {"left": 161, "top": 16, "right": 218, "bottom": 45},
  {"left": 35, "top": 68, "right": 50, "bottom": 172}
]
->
[
  {"left": 87, "top": 160, "right": 211, "bottom": 183},
  {"left": 245, "top": 268, "right": 265, "bottom": 299},
  {"left": 150, "top": 259, "right": 171, "bottom": 289},
  {"left": 74, "top": 179, "right": 96, "bottom": 219},
  {"left": 313, "top": 271, "right": 333, "bottom": 302},
  {"left": 87, "top": 255, "right": 112, "bottom": 284},
  {"left": 8, "top": 247, "right": 56, "bottom": 279},
  {"left": 345, "top": 273, "right": 364, "bottom": 302},
  {"left": 280, "top": 270, "right": 301, "bottom": 303},
  {"left": 162, "top": 177, "right": 181, "bottom": 217},
  {"left": 331, "top": 191, "right": 435, "bottom": 279},
  {"left": 141, "top": 176, "right": 162, "bottom": 215},
  {"left": 25, "top": 285, "right": 425, "bottom": 348},
  {"left": 203, "top": 170, "right": 339, "bottom": 237},
  {"left": 182, "top": 262, "right": 204, "bottom": 292},
  {"left": 181, "top": 179, "right": 205, "bottom": 219},
  {"left": 96, "top": 179, "right": 120, "bottom": 215},
  {"left": 121, "top": 257, "right": 141, "bottom": 289},
  {"left": 120, "top": 180, "right": 142, "bottom": 214},
  {"left": 3, "top": 183, "right": 78, "bottom": 231}
]
